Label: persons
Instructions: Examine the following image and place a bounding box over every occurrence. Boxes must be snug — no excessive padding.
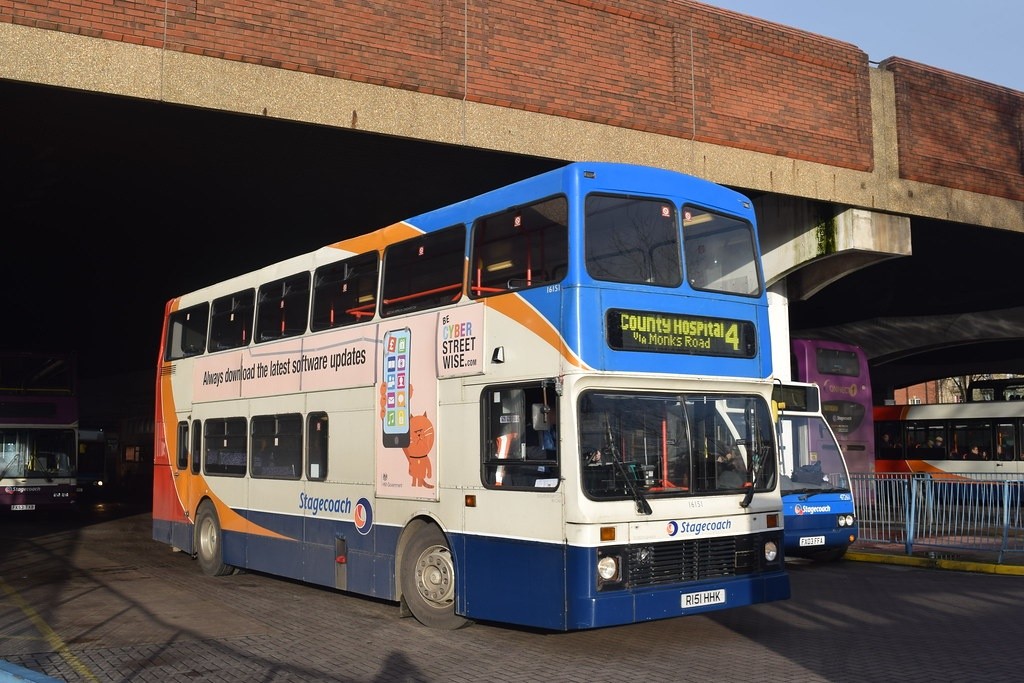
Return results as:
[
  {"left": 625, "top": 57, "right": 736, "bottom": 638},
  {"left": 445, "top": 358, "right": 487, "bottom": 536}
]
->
[
  {"left": 881, "top": 434, "right": 1024, "bottom": 461},
  {"left": 523, "top": 397, "right": 602, "bottom": 464},
  {"left": 683, "top": 439, "right": 732, "bottom": 487}
]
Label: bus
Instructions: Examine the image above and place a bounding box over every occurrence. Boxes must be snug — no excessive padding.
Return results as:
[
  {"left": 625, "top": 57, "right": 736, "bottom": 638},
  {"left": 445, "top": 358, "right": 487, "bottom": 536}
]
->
[
  {"left": 78, "top": 429, "right": 110, "bottom": 494},
  {"left": 677, "top": 379, "right": 858, "bottom": 561},
  {"left": 151, "top": 163, "right": 790, "bottom": 634},
  {"left": 791, "top": 339, "right": 878, "bottom": 508},
  {"left": 115, "top": 417, "right": 154, "bottom": 511},
  {"left": 1, "top": 349, "right": 87, "bottom": 522},
  {"left": 872, "top": 402, "right": 1024, "bottom": 507}
]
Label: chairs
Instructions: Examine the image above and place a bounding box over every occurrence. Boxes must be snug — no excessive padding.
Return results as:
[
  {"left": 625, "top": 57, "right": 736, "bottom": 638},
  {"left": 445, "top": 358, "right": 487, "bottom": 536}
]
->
[
  {"left": 186, "top": 322, "right": 332, "bottom": 353},
  {"left": 893, "top": 443, "right": 988, "bottom": 460},
  {"left": 190, "top": 449, "right": 293, "bottom": 475}
]
